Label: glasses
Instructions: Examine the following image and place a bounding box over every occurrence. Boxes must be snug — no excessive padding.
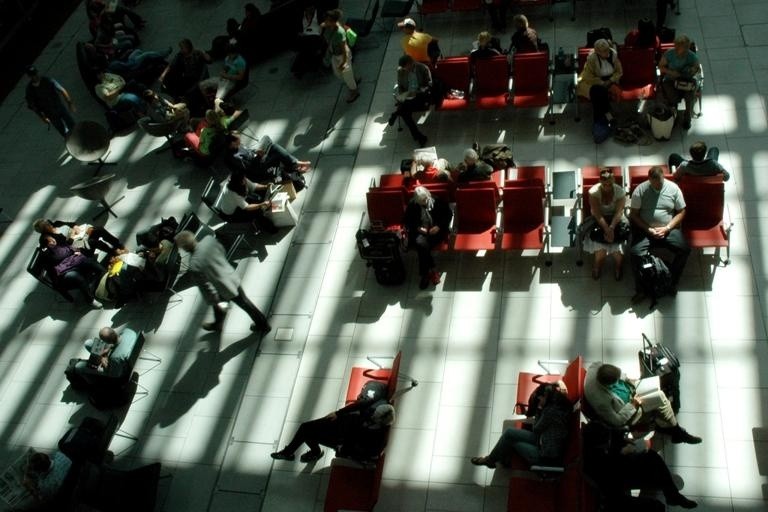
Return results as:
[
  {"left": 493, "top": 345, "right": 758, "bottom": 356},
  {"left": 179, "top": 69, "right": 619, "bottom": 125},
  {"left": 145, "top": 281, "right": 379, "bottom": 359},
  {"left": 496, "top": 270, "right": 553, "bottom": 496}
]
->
[{"left": 601, "top": 168, "right": 612, "bottom": 174}]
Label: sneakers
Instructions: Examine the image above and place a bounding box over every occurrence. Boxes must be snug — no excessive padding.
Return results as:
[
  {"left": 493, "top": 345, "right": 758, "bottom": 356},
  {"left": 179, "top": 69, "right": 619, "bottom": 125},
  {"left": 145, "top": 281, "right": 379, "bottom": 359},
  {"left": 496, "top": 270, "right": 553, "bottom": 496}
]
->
[
  {"left": 88, "top": 298, "right": 103, "bottom": 308},
  {"left": 251, "top": 323, "right": 272, "bottom": 331},
  {"left": 670, "top": 432, "right": 701, "bottom": 443},
  {"left": 346, "top": 90, "right": 359, "bottom": 103},
  {"left": 471, "top": 456, "right": 495, "bottom": 468},
  {"left": 202, "top": 323, "right": 221, "bottom": 331},
  {"left": 419, "top": 264, "right": 442, "bottom": 289},
  {"left": 271, "top": 450, "right": 295, "bottom": 461},
  {"left": 665, "top": 494, "right": 697, "bottom": 508},
  {"left": 389, "top": 112, "right": 397, "bottom": 125},
  {"left": 301, "top": 447, "right": 324, "bottom": 461},
  {"left": 683, "top": 121, "right": 691, "bottom": 129}
]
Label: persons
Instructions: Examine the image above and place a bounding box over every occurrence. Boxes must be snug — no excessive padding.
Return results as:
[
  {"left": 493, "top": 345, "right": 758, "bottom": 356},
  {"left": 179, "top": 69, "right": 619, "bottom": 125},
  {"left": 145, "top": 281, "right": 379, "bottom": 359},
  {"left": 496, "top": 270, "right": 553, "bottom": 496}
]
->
[
  {"left": 74, "top": 324, "right": 140, "bottom": 381},
  {"left": 472, "top": 356, "right": 700, "bottom": 510},
  {"left": 573, "top": 16, "right": 699, "bottom": 140},
  {"left": 24, "top": 67, "right": 80, "bottom": 140},
  {"left": 386, "top": 15, "right": 550, "bottom": 147},
  {"left": 400, "top": 142, "right": 492, "bottom": 290},
  {"left": 28, "top": 447, "right": 73, "bottom": 498},
  {"left": 34, "top": 220, "right": 126, "bottom": 257},
  {"left": 32, "top": 237, "right": 107, "bottom": 309},
  {"left": 270, "top": 400, "right": 394, "bottom": 464},
  {"left": 88, "top": 0, "right": 360, "bottom": 230},
  {"left": 583, "top": 142, "right": 731, "bottom": 309},
  {"left": 170, "top": 229, "right": 271, "bottom": 342}
]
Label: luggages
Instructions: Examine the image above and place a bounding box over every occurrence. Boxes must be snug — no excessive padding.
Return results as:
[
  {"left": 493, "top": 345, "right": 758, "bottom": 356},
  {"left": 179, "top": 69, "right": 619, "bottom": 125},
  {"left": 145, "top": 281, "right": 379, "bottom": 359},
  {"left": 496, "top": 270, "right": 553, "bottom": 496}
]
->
[
  {"left": 361, "top": 232, "right": 409, "bottom": 286},
  {"left": 639, "top": 333, "right": 680, "bottom": 412},
  {"left": 65, "top": 359, "right": 94, "bottom": 387}
]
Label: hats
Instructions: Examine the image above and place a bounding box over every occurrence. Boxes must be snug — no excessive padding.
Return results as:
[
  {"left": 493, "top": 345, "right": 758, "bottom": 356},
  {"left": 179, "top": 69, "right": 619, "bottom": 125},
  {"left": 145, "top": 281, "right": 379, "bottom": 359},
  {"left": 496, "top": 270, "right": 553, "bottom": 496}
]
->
[
  {"left": 26, "top": 65, "right": 38, "bottom": 77},
  {"left": 397, "top": 17, "right": 416, "bottom": 30}
]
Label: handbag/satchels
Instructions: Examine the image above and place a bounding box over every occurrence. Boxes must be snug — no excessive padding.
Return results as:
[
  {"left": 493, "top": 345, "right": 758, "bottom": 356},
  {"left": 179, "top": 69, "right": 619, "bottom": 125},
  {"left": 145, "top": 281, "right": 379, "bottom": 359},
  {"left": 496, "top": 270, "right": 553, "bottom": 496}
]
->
[
  {"left": 587, "top": 28, "right": 612, "bottom": 46},
  {"left": 266, "top": 168, "right": 305, "bottom": 228},
  {"left": 322, "top": 46, "right": 333, "bottom": 67},
  {"left": 646, "top": 102, "right": 677, "bottom": 142},
  {"left": 589, "top": 220, "right": 630, "bottom": 243},
  {"left": 362, "top": 233, "right": 397, "bottom": 263},
  {"left": 345, "top": 28, "right": 358, "bottom": 49},
  {"left": 673, "top": 76, "right": 696, "bottom": 91},
  {"left": 61, "top": 417, "right": 105, "bottom": 465},
  {"left": 482, "top": 144, "right": 513, "bottom": 168},
  {"left": 78, "top": 121, "right": 106, "bottom": 151},
  {"left": 614, "top": 112, "right": 651, "bottom": 146},
  {"left": 638, "top": 253, "right": 673, "bottom": 297},
  {"left": 358, "top": 380, "right": 388, "bottom": 404},
  {"left": 105, "top": 262, "right": 146, "bottom": 304}
]
